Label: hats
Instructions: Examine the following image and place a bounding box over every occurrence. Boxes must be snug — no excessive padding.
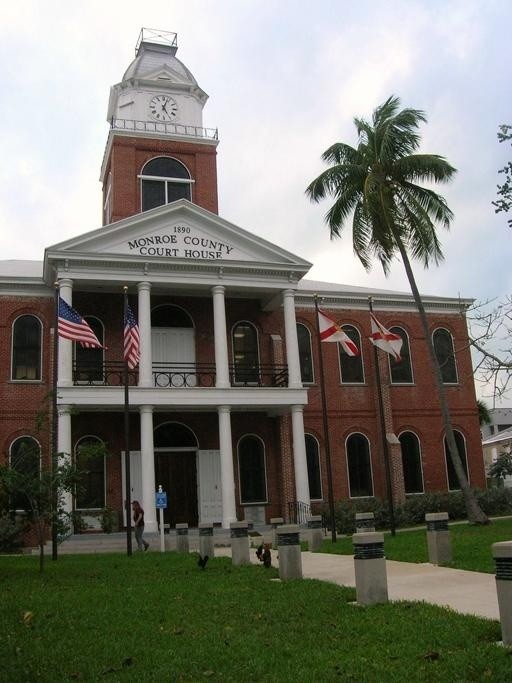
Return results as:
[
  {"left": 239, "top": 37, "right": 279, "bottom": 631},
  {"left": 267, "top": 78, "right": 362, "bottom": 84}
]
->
[{"left": 131, "top": 500, "right": 139, "bottom": 504}]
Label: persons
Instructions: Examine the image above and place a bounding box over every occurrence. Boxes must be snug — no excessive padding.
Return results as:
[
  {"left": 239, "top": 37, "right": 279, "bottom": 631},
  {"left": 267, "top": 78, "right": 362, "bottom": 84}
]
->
[{"left": 131, "top": 501, "right": 149, "bottom": 551}]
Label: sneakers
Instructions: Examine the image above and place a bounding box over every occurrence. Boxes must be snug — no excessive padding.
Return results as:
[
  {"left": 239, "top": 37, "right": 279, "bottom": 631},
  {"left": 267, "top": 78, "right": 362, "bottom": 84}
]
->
[{"left": 136, "top": 544, "right": 149, "bottom": 552}]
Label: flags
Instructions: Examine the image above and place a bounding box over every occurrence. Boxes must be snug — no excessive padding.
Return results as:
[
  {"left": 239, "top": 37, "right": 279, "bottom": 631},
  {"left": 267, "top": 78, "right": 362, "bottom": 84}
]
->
[
  {"left": 57, "top": 297, "right": 109, "bottom": 349},
  {"left": 317, "top": 309, "right": 360, "bottom": 358},
  {"left": 368, "top": 311, "right": 404, "bottom": 363},
  {"left": 124, "top": 295, "right": 139, "bottom": 370}
]
